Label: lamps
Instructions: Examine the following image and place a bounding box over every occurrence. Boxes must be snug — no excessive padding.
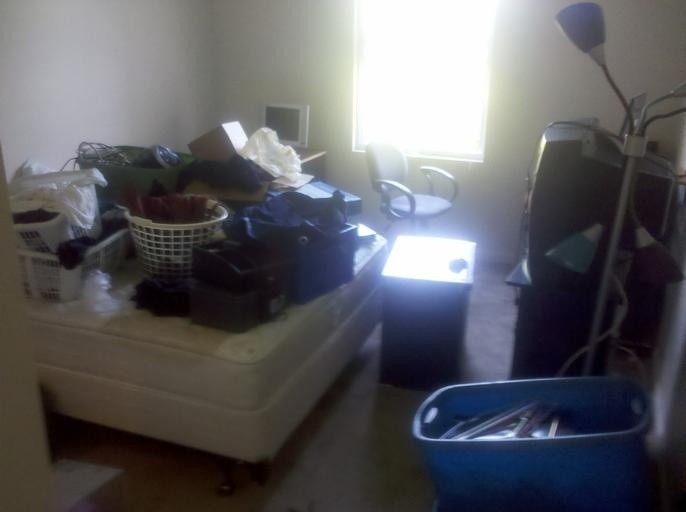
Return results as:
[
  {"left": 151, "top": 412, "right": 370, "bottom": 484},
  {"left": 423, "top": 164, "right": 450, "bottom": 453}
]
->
[{"left": 546, "top": 1, "right": 686, "bottom": 375}]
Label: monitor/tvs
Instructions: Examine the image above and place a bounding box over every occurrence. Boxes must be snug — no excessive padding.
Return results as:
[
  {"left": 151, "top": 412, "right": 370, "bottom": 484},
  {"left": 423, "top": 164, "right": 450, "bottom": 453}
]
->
[
  {"left": 524, "top": 116, "right": 675, "bottom": 290},
  {"left": 263, "top": 103, "right": 310, "bottom": 149}
]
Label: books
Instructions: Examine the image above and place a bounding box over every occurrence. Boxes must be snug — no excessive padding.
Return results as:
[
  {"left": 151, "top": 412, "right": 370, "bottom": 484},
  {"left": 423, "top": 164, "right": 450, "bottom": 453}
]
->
[
  {"left": 439, "top": 396, "right": 624, "bottom": 440},
  {"left": 179, "top": 175, "right": 270, "bottom": 205}
]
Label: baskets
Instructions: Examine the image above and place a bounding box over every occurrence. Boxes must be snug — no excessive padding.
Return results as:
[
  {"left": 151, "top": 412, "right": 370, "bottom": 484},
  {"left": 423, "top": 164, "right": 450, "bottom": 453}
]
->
[
  {"left": 124, "top": 195, "right": 229, "bottom": 289},
  {"left": 13, "top": 184, "right": 128, "bottom": 304}
]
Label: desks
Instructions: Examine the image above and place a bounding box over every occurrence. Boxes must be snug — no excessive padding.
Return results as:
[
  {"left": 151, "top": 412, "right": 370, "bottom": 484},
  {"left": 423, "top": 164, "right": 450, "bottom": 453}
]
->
[
  {"left": 379, "top": 235, "right": 477, "bottom": 393},
  {"left": 300, "top": 150, "right": 328, "bottom": 181},
  {"left": 505, "top": 260, "right": 623, "bottom": 380}
]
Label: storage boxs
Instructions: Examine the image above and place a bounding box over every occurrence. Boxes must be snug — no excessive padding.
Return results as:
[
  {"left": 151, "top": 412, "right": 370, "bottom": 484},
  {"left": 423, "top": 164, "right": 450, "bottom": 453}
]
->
[{"left": 411, "top": 377, "right": 653, "bottom": 512}]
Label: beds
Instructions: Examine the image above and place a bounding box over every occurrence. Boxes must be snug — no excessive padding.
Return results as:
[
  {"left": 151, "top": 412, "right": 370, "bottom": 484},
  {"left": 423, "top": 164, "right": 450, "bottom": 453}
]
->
[{"left": 30, "top": 224, "right": 388, "bottom": 487}]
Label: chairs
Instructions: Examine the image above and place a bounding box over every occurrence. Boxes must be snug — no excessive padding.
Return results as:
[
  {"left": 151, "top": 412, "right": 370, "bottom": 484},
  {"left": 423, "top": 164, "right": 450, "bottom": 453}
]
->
[{"left": 363, "top": 130, "right": 459, "bottom": 236}]
